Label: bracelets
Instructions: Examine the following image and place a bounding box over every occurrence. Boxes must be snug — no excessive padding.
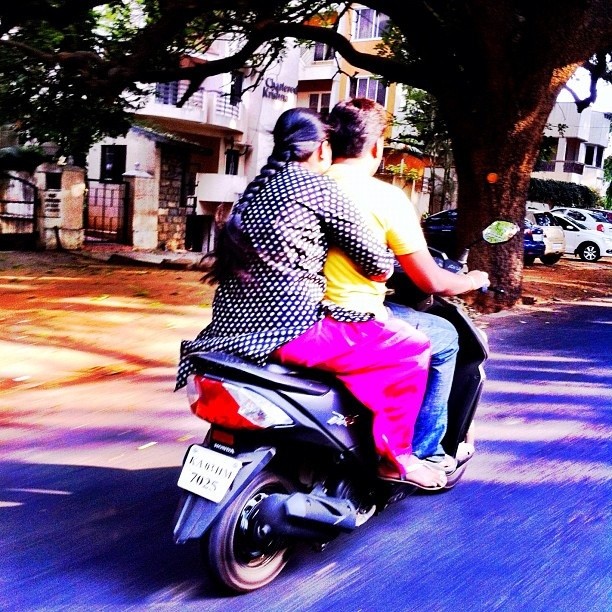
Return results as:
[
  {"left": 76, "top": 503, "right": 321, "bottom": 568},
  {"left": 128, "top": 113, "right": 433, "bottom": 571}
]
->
[{"left": 462, "top": 272, "right": 481, "bottom": 297}]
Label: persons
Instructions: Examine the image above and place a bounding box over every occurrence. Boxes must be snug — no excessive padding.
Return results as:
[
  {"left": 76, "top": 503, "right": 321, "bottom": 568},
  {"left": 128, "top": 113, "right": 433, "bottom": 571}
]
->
[
  {"left": 318, "top": 97, "right": 491, "bottom": 475},
  {"left": 173, "top": 107, "right": 448, "bottom": 493}
]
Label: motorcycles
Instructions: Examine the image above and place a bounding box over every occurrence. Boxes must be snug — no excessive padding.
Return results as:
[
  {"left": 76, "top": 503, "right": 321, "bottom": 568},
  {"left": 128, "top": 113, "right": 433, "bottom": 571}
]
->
[{"left": 170, "top": 246, "right": 492, "bottom": 594}]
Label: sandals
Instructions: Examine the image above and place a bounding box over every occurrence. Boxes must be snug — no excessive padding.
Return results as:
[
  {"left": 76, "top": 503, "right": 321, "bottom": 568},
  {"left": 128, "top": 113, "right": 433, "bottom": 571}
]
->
[
  {"left": 438, "top": 454, "right": 457, "bottom": 476},
  {"left": 377, "top": 456, "right": 447, "bottom": 490},
  {"left": 455, "top": 441, "right": 475, "bottom": 468}
]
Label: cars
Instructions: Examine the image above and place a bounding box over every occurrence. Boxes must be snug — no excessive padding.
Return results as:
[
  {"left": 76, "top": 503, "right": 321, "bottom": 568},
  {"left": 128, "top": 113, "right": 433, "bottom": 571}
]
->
[
  {"left": 570, "top": 210, "right": 612, "bottom": 234},
  {"left": 553, "top": 210, "right": 610, "bottom": 265},
  {"left": 527, "top": 208, "right": 563, "bottom": 261},
  {"left": 422, "top": 209, "right": 545, "bottom": 267}
]
